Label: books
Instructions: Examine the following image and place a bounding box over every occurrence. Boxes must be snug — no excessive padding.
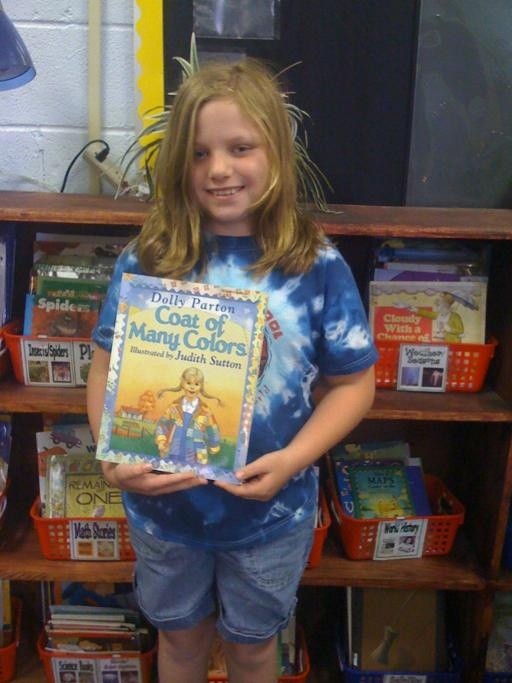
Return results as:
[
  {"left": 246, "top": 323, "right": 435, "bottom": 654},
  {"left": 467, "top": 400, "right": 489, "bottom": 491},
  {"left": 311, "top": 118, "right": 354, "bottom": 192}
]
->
[
  {"left": 0, "top": 227, "right": 299, "bottom": 682},
  {"left": 332, "top": 237, "right": 489, "bottom": 674}
]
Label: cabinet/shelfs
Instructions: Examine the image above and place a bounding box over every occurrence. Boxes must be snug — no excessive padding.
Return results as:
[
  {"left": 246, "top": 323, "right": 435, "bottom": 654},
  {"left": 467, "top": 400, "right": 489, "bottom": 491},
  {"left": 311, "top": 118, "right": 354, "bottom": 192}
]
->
[{"left": 0, "top": 190, "right": 511, "bottom": 682}]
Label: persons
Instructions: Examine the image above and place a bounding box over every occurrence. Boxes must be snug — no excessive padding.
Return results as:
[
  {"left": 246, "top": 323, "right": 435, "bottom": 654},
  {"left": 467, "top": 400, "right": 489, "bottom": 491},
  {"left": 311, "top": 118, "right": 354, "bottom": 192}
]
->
[{"left": 86, "top": 56, "right": 379, "bottom": 680}]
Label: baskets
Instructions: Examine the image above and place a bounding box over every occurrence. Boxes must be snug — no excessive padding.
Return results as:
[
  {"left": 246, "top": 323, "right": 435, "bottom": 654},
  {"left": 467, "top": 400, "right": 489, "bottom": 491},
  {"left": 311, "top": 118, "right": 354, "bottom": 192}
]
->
[
  {"left": 376, "top": 335, "right": 497, "bottom": 392},
  {"left": 209, "top": 621, "right": 311, "bottom": 683},
  {"left": 30, "top": 496, "right": 136, "bottom": 561},
  {"left": 306, "top": 471, "right": 465, "bottom": 568},
  {"left": 0, "top": 318, "right": 92, "bottom": 388},
  {"left": 0, "top": 596, "right": 158, "bottom": 683},
  {"left": 333, "top": 610, "right": 460, "bottom": 683}
]
